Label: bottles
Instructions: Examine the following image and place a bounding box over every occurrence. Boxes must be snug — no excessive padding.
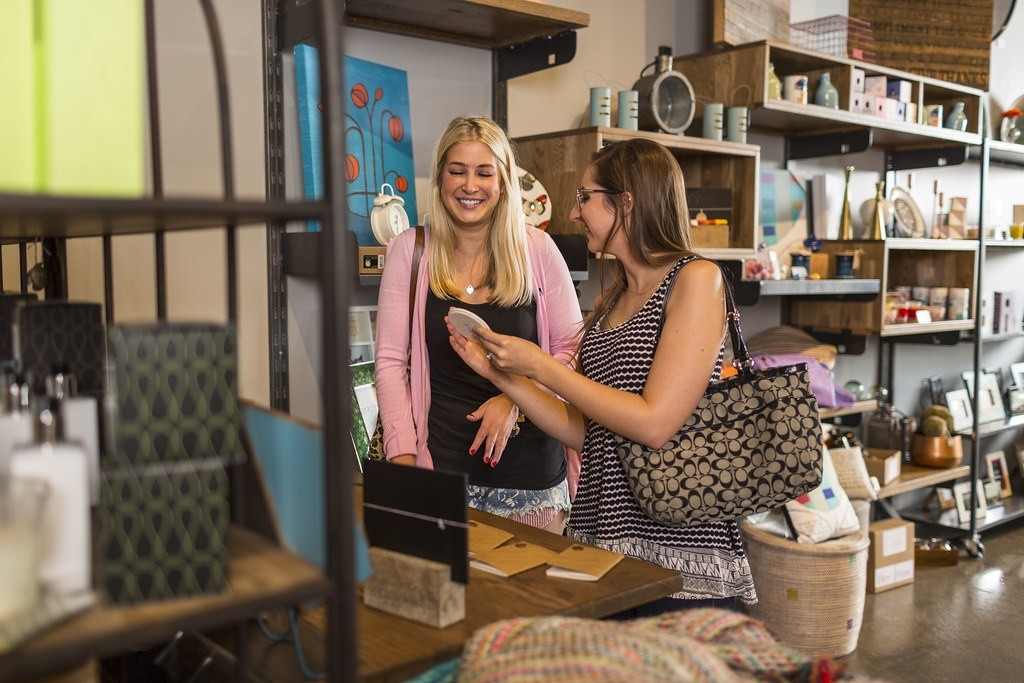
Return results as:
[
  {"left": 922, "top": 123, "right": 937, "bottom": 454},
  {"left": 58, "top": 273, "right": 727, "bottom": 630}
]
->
[
  {"left": 944, "top": 102, "right": 968, "bottom": 131},
  {"left": 1, "top": 371, "right": 101, "bottom": 618},
  {"left": 768, "top": 63, "right": 782, "bottom": 101},
  {"left": 813, "top": 72, "right": 839, "bottom": 110}
]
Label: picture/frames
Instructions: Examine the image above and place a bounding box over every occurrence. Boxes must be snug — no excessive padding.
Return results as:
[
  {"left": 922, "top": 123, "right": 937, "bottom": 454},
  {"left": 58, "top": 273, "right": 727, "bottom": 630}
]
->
[{"left": 928, "top": 364, "right": 1024, "bottom": 525}]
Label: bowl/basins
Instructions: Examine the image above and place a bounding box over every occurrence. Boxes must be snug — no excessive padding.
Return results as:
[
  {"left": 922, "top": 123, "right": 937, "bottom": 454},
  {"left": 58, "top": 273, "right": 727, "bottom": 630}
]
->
[{"left": 910, "top": 432, "right": 964, "bottom": 468}]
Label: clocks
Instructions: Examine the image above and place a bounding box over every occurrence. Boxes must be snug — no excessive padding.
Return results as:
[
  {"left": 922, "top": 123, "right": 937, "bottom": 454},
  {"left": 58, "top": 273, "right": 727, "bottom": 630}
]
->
[{"left": 370, "top": 183, "right": 410, "bottom": 246}]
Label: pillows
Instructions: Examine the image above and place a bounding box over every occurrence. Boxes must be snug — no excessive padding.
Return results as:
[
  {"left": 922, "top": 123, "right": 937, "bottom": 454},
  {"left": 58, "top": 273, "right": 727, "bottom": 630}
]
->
[
  {"left": 780, "top": 442, "right": 860, "bottom": 544},
  {"left": 828, "top": 446, "right": 879, "bottom": 501}
]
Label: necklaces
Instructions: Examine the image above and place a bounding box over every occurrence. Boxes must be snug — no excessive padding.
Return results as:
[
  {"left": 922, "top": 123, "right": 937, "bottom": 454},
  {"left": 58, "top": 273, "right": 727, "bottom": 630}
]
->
[{"left": 465, "top": 284, "right": 474, "bottom": 294}]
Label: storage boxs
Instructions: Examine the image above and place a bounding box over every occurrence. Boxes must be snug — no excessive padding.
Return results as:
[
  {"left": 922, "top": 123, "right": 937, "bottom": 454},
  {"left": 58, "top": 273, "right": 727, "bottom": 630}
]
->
[
  {"left": 8, "top": 301, "right": 242, "bottom": 607},
  {"left": 0, "top": 0, "right": 150, "bottom": 199},
  {"left": 689, "top": 223, "right": 729, "bottom": 250},
  {"left": 933, "top": 197, "right": 969, "bottom": 240},
  {"left": 861, "top": 446, "right": 902, "bottom": 486},
  {"left": 864, "top": 518, "right": 915, "bottom": 594},
  {"left": 848, "top": 70, "right": 918, "bottom": 124}
]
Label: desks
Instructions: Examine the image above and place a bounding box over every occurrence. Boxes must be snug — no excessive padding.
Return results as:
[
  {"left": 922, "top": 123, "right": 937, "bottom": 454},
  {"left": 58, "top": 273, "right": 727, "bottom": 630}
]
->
[{"left": 99, "top": 505, "right": 684, "bottom": 683}]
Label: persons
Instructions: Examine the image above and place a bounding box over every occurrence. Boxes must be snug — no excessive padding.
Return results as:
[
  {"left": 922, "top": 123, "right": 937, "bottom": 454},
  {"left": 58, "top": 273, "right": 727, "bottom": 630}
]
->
[
  {"left": 374, "top": 115, "right": 582, "bottom": 536},
  {"left": 444, "top": 138, "right": 758, "bottom": 621}
]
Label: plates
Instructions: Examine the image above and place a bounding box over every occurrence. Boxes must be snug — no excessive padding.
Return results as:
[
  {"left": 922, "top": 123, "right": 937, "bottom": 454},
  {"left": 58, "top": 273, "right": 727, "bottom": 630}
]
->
[{"left": 890, "top": 186, "right": 927, "bottom": 237}]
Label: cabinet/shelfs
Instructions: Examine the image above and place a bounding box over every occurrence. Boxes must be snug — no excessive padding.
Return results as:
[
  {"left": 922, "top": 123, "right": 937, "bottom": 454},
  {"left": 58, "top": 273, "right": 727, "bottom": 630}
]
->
[
  {"left": 0, "top": 1, "right": 355, "bottom": 683},
  {"left": 261, "top": 0, "right": 589, "bottom": 415},
  {"left": 508, "top": 39, "right": 1024, "bottom": 561}
]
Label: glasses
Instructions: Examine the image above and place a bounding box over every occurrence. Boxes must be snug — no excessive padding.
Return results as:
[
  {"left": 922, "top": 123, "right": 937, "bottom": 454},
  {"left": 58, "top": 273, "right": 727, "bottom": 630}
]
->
[{"left": 575, "top": 187, "right": 626, "bottom": 210}]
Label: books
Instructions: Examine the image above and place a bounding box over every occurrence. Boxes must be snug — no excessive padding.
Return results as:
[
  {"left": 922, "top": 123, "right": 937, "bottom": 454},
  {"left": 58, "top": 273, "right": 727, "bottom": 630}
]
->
[
  {"left": 469, "top": 541, "right": 557, "bottom": 577},
  {"left": 465, "top": 520, "right": 514, "bottom": 558},
  {"left": 546, "top": 544, "right": 625, "bottom": 581}
]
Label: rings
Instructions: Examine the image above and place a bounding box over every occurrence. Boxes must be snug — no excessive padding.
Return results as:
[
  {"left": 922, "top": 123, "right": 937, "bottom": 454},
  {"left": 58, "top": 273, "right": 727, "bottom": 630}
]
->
[{"left": 486, "top": 353, "right": 492, "bottom": 359}]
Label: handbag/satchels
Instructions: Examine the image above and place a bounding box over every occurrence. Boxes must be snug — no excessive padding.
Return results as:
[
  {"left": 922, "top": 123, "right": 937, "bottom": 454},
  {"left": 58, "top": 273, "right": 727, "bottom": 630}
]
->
[
  {"left": 365, "top": 353, "right": 418, "bottom": 461},
  {"left": 614, "top": 258, "right": 823, "bottom": 527}
]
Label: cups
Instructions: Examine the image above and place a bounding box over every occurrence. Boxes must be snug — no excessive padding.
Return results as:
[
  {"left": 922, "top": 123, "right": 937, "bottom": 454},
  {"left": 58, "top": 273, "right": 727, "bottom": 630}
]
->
[
  {"left": 702, "top": 102, "right": 723, "bottom": 142},
  {"left": 617, "top": 91, "right": 638, "bottom": 131},
  {"left": 728, "top": 106, "right": 748, "bottom": 144},
  {"left": 791, "top": 254, "right": 810, "bottom": 277},
  {"left": 885, "top": 286, "right": 969, "bottom": 325},
  {"left": 927, "top": 105, "right": 943, "bottom": 128},
  {"left": 590, "top": 87, "right": 611, "bottom": 127},
  {"left": 835, "top": 252, "right": 854, "bottom": 278},
  {"left": 785, "top": 74, "right": 808, "bottom": 104}
]
